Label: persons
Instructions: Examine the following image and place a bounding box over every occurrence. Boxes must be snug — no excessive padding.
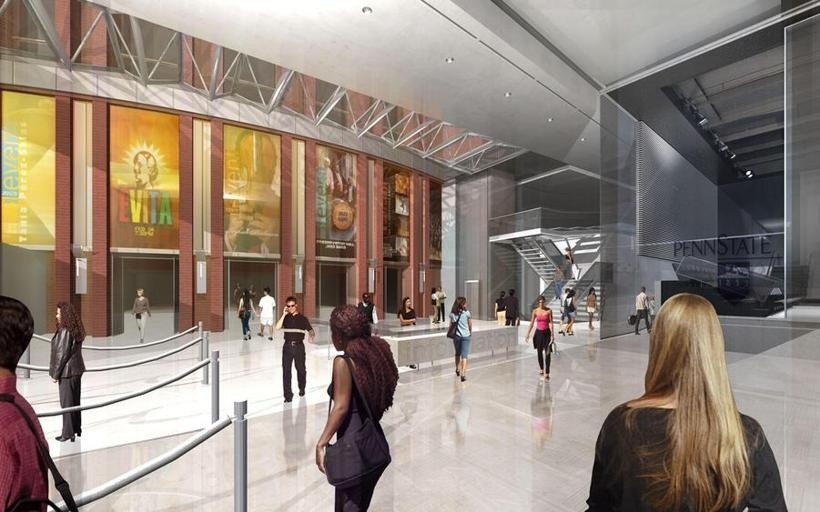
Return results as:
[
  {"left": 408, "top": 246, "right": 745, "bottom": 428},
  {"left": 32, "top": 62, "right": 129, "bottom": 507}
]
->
[
  {"left": 586, "top": 287, "right": 598, "bottom": 332},
  {"left": 397, "top": 296, "right": 417, "bottom": 369},
  {"left": 313, "top": 152, "right": 357, "bottom": 208},
  {"left": 583, "top": 292, "right": 788, "bottom": 512},
  {"left": 223, "top": 128, "right": 282, "bottom": 254},
  {"left": 523, "top": 295, "right": 554, "bottom": 382},
  {"left": 448, "top": 296, "right": 473, "bottom": 382},
  {"left": 634, "top": 285, "right": 657, "bottom": 335},
  {"left": 430, "top": 286, "right": 447, "bottom": 324},
  {"left": 49, "top": 300, "right": 85, "bottom": 442},
  {"left": 315, "top": 302, "right": 400, "bottom": 512},
  {"left": 233, "top": 282, "right": 276, "bottom": 342},
  {"left": 133, "top": 149, "right": 159, "bottom": 189},
  {"left": 494, "top": 289, "right": 519, "bottom": 326},
  {"left": 354, "top": 292, "right": 379, "bottom": 337},
  {"left": 391, "top": 172, "right": 410, "bottom": 258},
  {"left": 275, "top": 296, "right": 315, "bottom": 403},
  {"left": 131, "top": 287, "right": 152, "bottom": 345},
  {"left": 554, "top": 245, "right": 579, "bottom": 336},
  {"left": 0, "top": 294, "right": 50, "bottom": 512}
]
